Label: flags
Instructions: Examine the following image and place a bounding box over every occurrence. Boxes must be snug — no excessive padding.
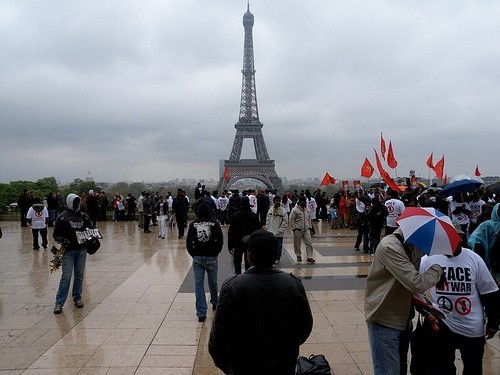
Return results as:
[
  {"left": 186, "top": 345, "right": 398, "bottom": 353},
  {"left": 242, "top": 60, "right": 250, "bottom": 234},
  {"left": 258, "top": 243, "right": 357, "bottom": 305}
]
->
[
  {"left": 223, "top": 166, "right": 229, "bottom": 182},
  {"left": 474, "top": 165, "right": 481, "bottom": 177},
  {"left": 360, "top": 132, "right": 401, "bottom": 187},
  {"left": 320, "top": 172, "right": 336, "bottom": 185},
  {"left": 426, "top": 152, "right": 447, "bottom": 186}
]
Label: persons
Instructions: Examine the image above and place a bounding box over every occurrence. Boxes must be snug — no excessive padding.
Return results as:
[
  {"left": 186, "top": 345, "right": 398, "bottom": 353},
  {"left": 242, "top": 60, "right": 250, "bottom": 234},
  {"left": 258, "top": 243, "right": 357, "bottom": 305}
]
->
[
  {"left": 321, "top": 187, "right": 500, "bottom": 285},
  {"left": 207, "top": 229, "right": 313, "bottom": 375},
  {"left": 26, "top": 198, "right": 49, "bottom": 250},
  {"left": 264, "top": 194, "right": 289, "bottom": 266},
  {"left": 288, "top": 194, "right": 315, "bottom": 263},
  {"left": 51, "top": 193, "right": 95, "bottom": 314},
  {"left": 418, "top": 220, "right": 500, "bottom": 375},
  {"left": 194, "top": 183, "right": 321, "bottom": 234},
  {"left": 364, "top": 223, "right": 449, "bottom": 375},
  {"left": 227, "top": 196, "right": 262, "bottom": 275},
  {"left": 16, "top": 185, "right": 191, "bottom": 239},
  {"left": 186, "top": 202, "right": 224, "bottom": 322}
]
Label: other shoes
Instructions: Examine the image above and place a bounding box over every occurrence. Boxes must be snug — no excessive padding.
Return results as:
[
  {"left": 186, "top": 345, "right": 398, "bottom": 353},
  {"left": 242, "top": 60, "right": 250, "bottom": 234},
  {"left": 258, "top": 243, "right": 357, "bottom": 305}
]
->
[
  {"left": 158, "top": 235, "right": 161, "bottom": 237},
  {"left": 144, "top": 231, "right": 151, "bottom": 233},
  {"left": 275, "top": 260, "right": 279, "bottom": 264},
  {"left": 212, "top": 305, "right": 216, "bottom": 311},
  {"left": 42, "top": 243, "right": 47, "bottom": 249},
  {"left": 297, "top": 256, "right": 302, "bottom": 261},
  {"left": 161, "top": 237, "right": 164, "bottom": 239},
  {"left": 199, "top": 316, "right": 206, "bottom": 322},
  {"left": 33, "top": 247, "right": 39, "bottom": 250},
  {"left": 307, "top": 258, "right": 315, "bottom": 262}
]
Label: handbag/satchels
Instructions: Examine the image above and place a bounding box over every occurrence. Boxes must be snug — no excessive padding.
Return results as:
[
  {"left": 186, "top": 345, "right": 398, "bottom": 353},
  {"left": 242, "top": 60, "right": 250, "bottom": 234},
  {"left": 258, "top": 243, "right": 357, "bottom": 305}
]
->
[
  {"left": 87, "top": 237, "right": 101, "bottom": 255},
  {"left": 409, "top": 312, "right": 450, "bottom": 375},
  {"left": 295, "top": 354, "right": 332, "bottom": 375}
]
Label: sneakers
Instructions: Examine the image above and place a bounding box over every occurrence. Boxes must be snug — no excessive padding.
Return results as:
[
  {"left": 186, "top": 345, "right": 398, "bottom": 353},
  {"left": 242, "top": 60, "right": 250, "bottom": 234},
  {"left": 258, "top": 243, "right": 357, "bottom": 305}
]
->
[
  {"left": 54, "top": 304, "right": 62, "bottom": 314},
  {"left": 74, "top": 298, "right": 84, "bottom": 308}
]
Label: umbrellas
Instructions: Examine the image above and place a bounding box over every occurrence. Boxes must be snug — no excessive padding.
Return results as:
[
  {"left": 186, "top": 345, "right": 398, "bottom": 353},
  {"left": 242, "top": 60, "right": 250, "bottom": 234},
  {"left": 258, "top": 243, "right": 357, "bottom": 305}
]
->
[
  {"left": 441, "top": 173, "right": 483, "bottom": 198},
  {"left": 396, "top": 207, "right": 462, "bottom": 259}
]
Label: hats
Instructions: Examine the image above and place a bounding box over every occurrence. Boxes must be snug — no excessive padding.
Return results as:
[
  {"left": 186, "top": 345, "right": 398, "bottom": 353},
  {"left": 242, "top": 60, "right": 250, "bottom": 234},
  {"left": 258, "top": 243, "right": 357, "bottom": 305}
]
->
[
  {"left": 299, "top": 197, "right": 306, "bottom": 202},
  {"left": 239, "top": 197, "right": 250, "bottom": 208}
]
